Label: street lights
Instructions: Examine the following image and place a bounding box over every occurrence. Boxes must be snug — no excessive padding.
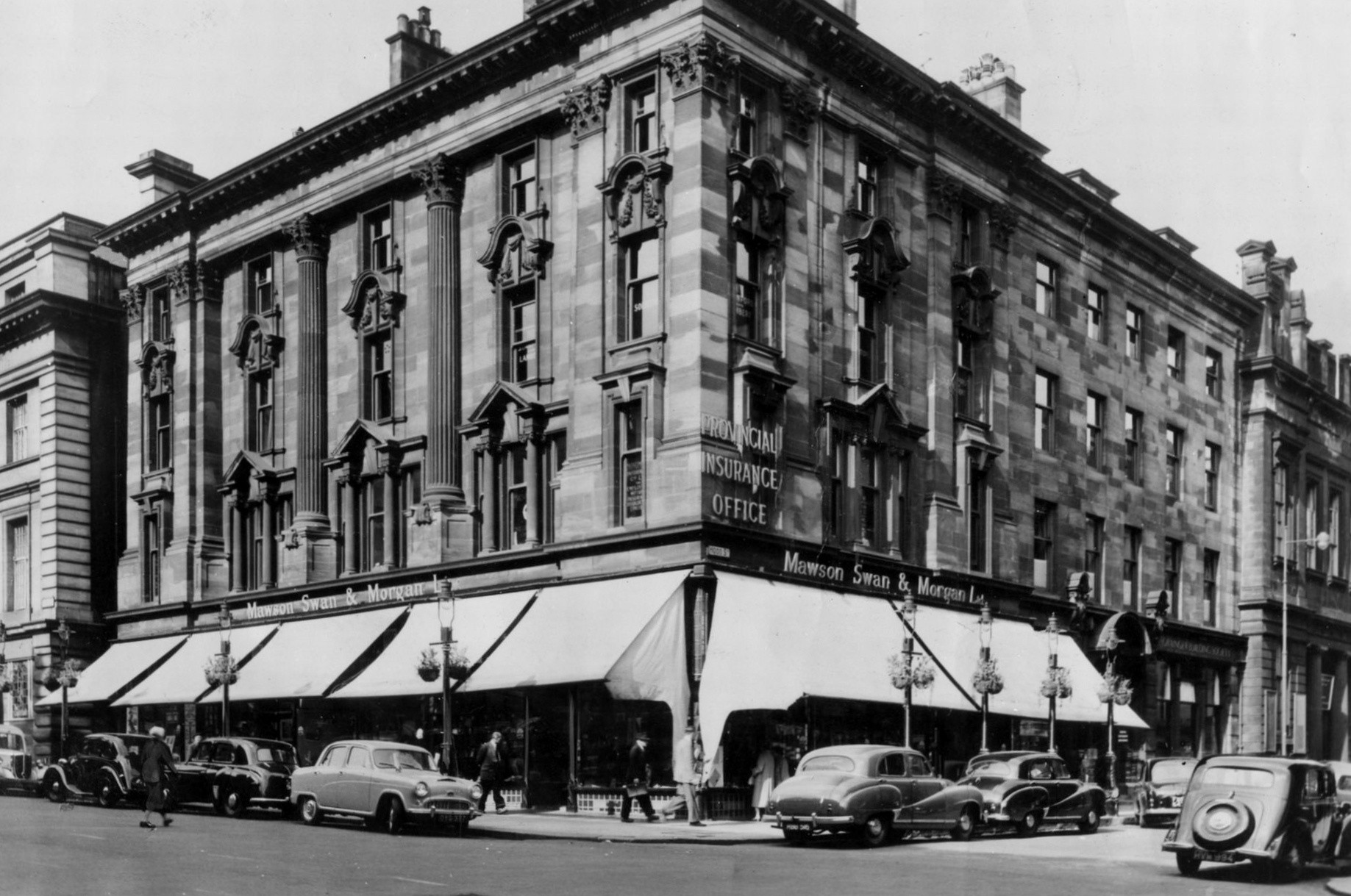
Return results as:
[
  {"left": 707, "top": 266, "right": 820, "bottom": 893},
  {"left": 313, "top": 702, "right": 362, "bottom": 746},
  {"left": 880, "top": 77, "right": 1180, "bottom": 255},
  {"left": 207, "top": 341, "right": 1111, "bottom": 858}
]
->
[
  {"left": 203, "top": 600, "right": 240, "bottom": 740},
  {"left": 972, "top": 600, "right": 1004, "bottom": 757},
  {"left": 888, "top": 589, "right": 935, "bottom": 748},
  {"left": 1098, "top": 626, "right": 1134, "bottom": 821},
  {"left": 1040, "top": 612, "right": 1073, "bottom": 756},
  {"left": 417, "top": 575, "right": 472, "bottom": 783},
  {"left": 44, "top": 618, "right": 92, "bottom": 760},
  {"left": 1276, "top": 526, "right": 1330, "bottom": 757}
]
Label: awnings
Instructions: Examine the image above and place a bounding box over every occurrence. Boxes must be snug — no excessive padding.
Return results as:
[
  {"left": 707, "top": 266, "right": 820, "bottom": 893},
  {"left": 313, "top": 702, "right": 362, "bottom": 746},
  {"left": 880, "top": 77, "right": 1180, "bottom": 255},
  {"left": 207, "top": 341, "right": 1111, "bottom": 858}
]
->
[{"left": 38, "top": 564, "right": 1152, "bottom": 732}]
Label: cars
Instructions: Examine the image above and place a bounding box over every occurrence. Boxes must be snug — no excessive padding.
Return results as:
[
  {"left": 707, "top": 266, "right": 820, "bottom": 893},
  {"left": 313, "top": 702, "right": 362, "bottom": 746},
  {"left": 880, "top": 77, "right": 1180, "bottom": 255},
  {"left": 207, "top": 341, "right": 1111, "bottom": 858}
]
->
[
  {"left": 158, "top": 736, "right": 303, "bottom": 817},
  {"left": 955, "top": 751, "right": 1107, "bottom": 837},
  {"left": 1308, "top": 760, "right": 1351, "bottom": 805},
  {"left": 1135, "top": 756, "right": 1204, "bottom": 829},
  {"left": 0, "top": 722, "right": 47, "bottom": 794},
  {"left": 769, "top": 745, "right": 984, "bottom": 844},
  {"left": 43, "top": 733, "right": 176, "bottom": 809},
  {"left": 291, "top": 739, "right": 482, "bottom": 833},
  {"left": 1162, "top": 751, "right": 1351, "bottom": 884}
]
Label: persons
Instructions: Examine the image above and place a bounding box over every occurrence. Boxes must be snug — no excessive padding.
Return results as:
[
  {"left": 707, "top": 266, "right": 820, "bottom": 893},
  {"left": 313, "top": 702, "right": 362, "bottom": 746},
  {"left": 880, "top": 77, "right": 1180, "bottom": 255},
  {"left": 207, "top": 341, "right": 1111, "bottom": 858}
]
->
[
  {"left": 619, "top": 731, "right": 658, "bottom": 823},
  {"left": 662, "top": 729, "right": 707, "bottom": 829},
  {"left": 133, "top": 724, "right": 188, "bottom": 831},
  {"left": 748, "top": 739, "right": 779, "bottom": 822},
  {"left": 474, "top": 731, "right": 509, "bottom": 815}
]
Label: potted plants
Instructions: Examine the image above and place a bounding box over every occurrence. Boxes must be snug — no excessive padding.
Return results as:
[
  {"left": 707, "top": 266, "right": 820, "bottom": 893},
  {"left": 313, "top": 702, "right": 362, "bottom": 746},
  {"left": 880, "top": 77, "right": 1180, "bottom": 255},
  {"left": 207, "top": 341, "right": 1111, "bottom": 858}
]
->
[
  {"left": 205, "top": 655, "right": 238, "bottom": 687},
  {"left": 448, "top": 644, "right": 469, "bottom": 681},
  {"left": 885, "top": 649, "right": 1134, "bottom": 705},
  {"left": 418, "top": 647, "right": 441, "bottom": 682},
  {"left": 57, "top": 659, "right": 82, "bottom": 687}
]
